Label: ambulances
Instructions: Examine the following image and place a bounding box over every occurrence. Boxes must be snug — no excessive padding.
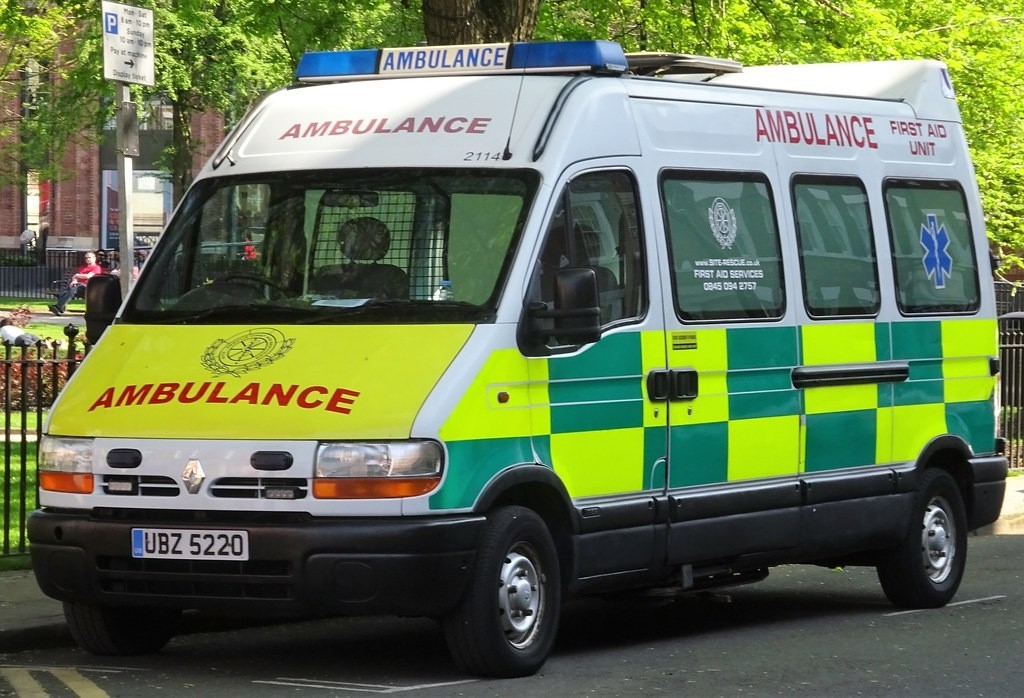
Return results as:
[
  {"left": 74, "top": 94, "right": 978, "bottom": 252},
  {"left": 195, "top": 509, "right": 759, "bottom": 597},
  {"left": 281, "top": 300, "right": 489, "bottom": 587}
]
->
[{"left": 27, "top": 39, "right": 1010, "bottom": 679}]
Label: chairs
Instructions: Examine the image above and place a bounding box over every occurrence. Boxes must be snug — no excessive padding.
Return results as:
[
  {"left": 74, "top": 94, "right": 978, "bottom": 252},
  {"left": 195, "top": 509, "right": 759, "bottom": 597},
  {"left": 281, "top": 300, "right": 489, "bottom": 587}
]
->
[
  {"left": 312, "top": 218, "right": 407, "bottom": 301},
  {"left": 552, "top": 226, "right": 623, "bottom": 324}
]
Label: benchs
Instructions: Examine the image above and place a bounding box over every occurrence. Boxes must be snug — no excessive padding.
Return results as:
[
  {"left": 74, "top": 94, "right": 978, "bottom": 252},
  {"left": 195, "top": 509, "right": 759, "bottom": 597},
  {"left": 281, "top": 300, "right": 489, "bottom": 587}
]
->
[{"left": 45, "top": 266, "right": 112, "bottom": 311}]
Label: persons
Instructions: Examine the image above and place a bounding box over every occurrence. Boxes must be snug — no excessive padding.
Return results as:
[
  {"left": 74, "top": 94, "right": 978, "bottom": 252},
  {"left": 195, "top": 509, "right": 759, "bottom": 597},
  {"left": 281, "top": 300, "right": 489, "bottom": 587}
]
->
[
  {"left": 243, "top": 238, "right": 256, "bottom": 260},
  {"left": 47, "top": 248, "right": 147, "bottom": 316}
]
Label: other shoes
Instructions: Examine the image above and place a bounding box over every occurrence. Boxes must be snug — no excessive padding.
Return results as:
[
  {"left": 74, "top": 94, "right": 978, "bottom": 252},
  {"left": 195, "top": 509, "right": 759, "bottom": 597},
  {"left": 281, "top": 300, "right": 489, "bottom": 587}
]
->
[
  {"left": 49, "top": 305, "right": 62, "bottom": 316},
  {"left": 56, "top": 304, "right": 63, "bottom": 313}
]
